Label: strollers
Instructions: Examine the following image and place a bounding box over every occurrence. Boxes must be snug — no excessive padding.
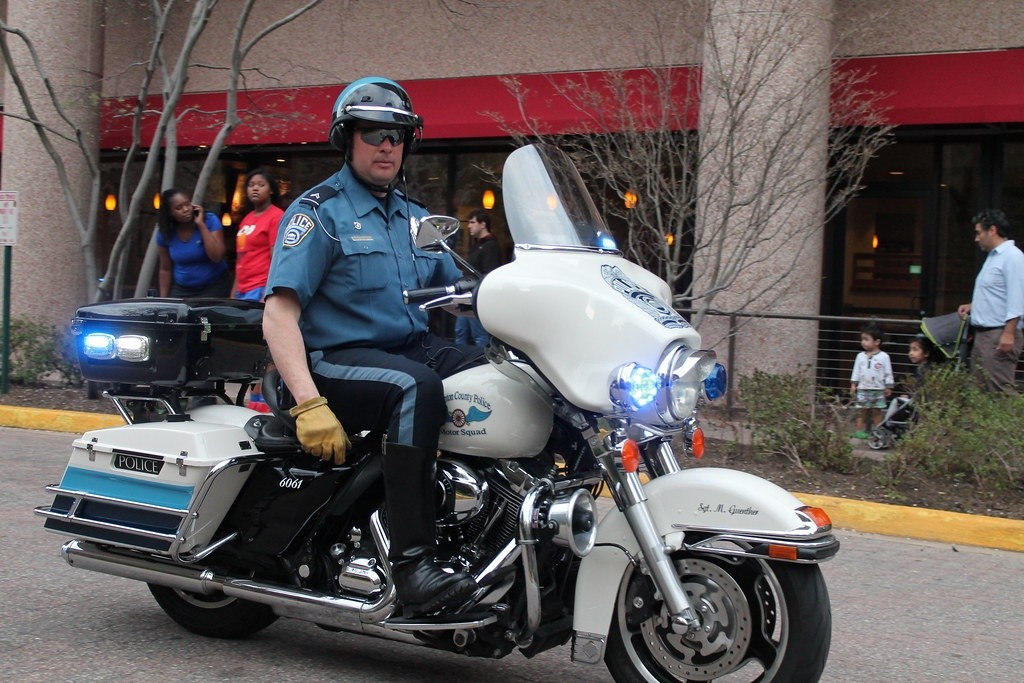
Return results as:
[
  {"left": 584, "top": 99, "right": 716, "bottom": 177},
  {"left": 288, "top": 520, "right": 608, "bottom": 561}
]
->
[{"left": 868, "top": 309, "right": 975, "bottom": 452}]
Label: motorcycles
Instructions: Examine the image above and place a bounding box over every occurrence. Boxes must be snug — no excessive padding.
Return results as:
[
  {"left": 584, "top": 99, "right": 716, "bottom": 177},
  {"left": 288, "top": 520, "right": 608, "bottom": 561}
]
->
[{"left": 34, "top": 141, "right": 840, "bottom": 683}]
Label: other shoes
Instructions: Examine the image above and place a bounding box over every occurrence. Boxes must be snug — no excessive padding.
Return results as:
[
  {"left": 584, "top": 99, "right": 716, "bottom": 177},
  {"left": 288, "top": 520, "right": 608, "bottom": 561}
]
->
[{"left": 854, "top": 430, "right": 869, "bottom": 439}]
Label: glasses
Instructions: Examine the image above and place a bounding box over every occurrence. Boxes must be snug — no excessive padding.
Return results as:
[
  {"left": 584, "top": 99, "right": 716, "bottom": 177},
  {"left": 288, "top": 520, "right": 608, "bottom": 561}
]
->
[{"left": 351, "top": 125, "right": 407, "bottom": 146}]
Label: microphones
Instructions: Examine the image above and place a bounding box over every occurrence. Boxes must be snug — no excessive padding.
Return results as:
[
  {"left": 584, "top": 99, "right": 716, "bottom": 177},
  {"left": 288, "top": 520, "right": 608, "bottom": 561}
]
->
[{"left": 416, "top": 115, "right": 424, "bottom": 147}]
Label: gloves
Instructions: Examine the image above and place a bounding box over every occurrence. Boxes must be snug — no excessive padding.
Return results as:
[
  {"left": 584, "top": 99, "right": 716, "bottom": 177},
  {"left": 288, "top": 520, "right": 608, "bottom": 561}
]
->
[{"left": 289, "top": 397, "right": 352, "bottom": 466}]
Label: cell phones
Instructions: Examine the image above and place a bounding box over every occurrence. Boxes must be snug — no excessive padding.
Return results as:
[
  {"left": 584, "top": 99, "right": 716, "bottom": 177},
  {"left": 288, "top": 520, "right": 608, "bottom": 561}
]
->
[{"left": 191, "top": 206, "right": 199, "bottom": 216}]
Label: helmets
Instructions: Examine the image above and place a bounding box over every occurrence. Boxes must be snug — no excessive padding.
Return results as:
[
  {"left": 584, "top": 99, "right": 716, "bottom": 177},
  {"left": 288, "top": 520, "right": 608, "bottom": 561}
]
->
[{"left": 329, "top": 76, "right": 422, "bottom": 151}]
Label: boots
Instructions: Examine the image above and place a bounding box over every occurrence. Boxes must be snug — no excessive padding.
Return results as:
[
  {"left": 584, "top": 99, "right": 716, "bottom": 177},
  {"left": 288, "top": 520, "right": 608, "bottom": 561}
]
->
[{"left": 382, "top": 437, "right": 478, "bottom": 620}]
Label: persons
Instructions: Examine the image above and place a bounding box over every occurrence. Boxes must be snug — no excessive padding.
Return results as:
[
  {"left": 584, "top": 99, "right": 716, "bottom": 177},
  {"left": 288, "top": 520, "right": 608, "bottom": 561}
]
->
[
  {"left": 156, "top": 187, "right": 233, "bottom": 406},
  {"left": 849, "top": 325, "right": 895, "bottom": 434},
  {"left": 454, "top": 209, "right": 502, "bottom": 347},
  {"left": 261, "top": 76, "right": 474, "bottom": 621},
  {"left": 229, "top": 164, "right": 290, "bottom": 413},
  {"left": 957, "top": 209, "right": 1024, "bottom": 397},
  {"left": 884, "top": 337, "right": 934, "bottom": 422}
]
